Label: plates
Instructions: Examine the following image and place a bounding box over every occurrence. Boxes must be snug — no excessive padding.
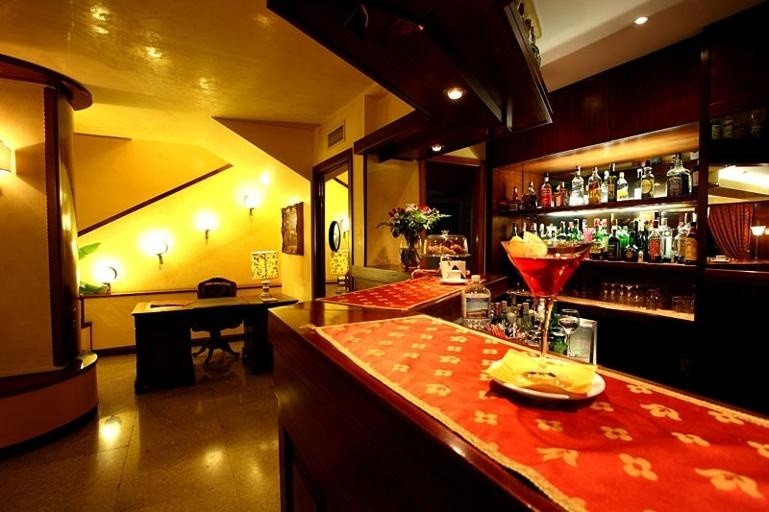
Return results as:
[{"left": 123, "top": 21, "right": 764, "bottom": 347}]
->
[
  {"left": 440, "top": 278, "right": 468, "bottom": 284},
  {"left": 484, "top": 357, "right": 606, "bottom": 401}
]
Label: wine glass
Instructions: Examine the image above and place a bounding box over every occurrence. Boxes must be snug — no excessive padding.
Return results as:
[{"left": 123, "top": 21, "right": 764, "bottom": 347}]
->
[
  {"left": 599, "top": 281, "right": 663, "bottom": 311},
  {"left": 560, "top": 308, "right": 580, "bottom": 358}
]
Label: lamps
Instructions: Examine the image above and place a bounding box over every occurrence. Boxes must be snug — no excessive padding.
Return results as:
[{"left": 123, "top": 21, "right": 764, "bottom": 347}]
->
[
  {"left": 198, "top": 214, "right": 216, "bottom": 242},
  {"left": 0, "top": 142, "right": 15, "bottom": 174},
  {"left": 244, "top": 191, "right": 259, "bottom": 215},
  {"left": 103, "top": 267, "right": 115, "bottom": 281},
  {"left": 153, "top": 241, "right": 169, "bottom": 268},
  {"left": 750, "top": 225, "right": 765, "bottom": 260}
]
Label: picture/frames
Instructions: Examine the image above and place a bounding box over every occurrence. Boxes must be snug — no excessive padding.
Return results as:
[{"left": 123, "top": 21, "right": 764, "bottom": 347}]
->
[{"left": 280, "top": 202, "right": 304, "bottom": 255}]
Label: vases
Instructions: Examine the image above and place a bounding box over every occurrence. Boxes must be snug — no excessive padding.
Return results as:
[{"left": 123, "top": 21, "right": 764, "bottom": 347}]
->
[{"left": 398, "top": 233, "right": 423, "bottom": 270}]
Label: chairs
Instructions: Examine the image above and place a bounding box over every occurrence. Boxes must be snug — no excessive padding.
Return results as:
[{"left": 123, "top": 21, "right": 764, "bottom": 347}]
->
[{"left": 191, "top": 277, "right": 241, "bottom": 368}]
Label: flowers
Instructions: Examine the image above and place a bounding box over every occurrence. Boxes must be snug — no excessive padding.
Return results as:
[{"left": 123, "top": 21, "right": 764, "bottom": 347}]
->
[{"left": 376, "top": 203, "right": 452, "bottom": 241}]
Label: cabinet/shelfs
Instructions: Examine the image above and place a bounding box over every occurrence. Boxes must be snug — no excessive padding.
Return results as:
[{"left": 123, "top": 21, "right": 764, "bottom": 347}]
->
[
  {"left": 129, "top": 292, "right": 299, "bottom": 396},
  {"left": 706, "top": 108, "right": 769, "bottom": 273},
  {"left": 490, "top": 121, "right": 701, "bottom": 322}
]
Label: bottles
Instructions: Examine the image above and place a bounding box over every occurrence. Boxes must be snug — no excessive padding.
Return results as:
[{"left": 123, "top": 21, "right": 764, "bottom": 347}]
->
[
  {"left": 499, "top": 223, "right": 509, "bottom": 270},
  {"left": 461, "top": 275, "right": 567, "bottom": 355},
  {"left": 509, "top": 152, "right": 700, "bottom": 265},
  {"left": 710, "top": 108, "right": 763, "bottom": 140},
  {"left": 497, "top": 190, "right": 509, "bottom": 212}
]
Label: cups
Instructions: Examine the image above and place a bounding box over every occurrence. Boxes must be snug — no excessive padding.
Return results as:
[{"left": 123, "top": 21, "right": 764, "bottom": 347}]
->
[
  {"left": 447, "top": 270, "right": 461, "bottom": 282},
  {"left": 671, "top": 295, "right": 695, "bottom": 313}
]
